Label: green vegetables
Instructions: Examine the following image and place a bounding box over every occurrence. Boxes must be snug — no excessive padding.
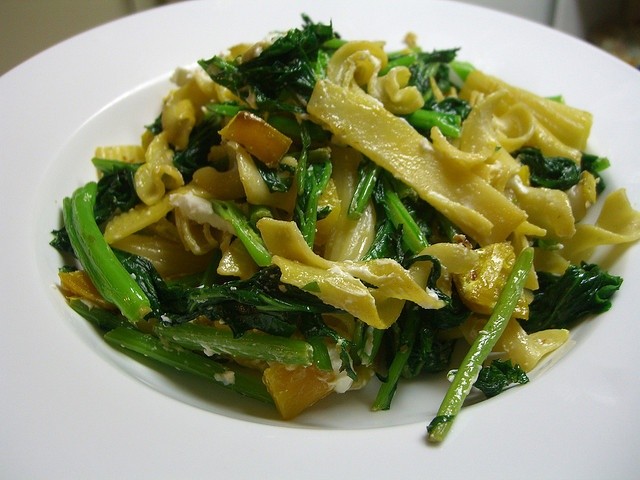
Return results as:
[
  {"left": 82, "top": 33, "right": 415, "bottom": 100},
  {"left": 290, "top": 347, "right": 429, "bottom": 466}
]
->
[{"left": 49, "top": 12, "right": 640, "bottom": 443}]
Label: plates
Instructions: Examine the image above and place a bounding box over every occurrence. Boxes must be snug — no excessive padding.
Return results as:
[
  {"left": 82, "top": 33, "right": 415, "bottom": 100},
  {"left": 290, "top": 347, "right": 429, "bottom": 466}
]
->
[{"left": 1, "top": 0, "right": 639, "bottom": 476}]
